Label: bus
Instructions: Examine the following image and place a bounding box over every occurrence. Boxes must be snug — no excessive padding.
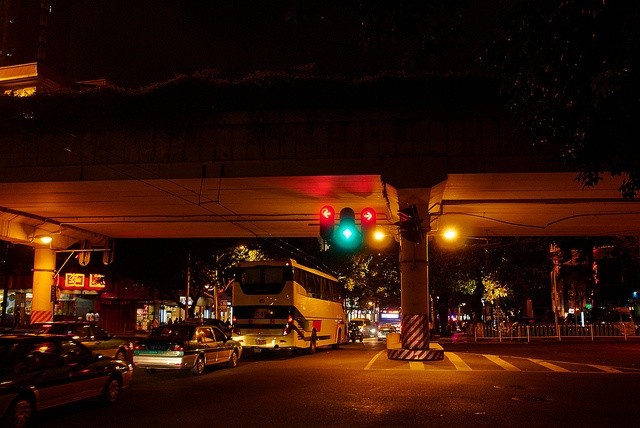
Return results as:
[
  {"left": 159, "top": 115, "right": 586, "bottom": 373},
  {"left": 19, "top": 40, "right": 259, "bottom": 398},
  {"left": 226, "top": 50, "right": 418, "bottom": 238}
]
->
[
  {"left": 231, "top": 257, "right": 349, "bottom": 354},
  {"left": 379, "top": 307, "right": 401, "bottom": 334}
]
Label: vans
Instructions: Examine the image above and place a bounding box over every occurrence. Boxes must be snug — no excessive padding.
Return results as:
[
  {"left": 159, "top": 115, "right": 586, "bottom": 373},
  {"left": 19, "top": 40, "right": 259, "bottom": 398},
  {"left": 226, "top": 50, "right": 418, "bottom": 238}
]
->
[{"left": 350, "top": 318, "right": 370, "bottom": 338}]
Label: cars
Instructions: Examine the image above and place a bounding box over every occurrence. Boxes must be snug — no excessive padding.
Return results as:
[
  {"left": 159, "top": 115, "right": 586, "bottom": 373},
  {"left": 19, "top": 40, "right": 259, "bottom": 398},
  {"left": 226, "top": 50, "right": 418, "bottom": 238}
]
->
[
  {"left": 348, "top": 322, "right": 363, "bottom": 343},
  {"left": 377, "top": 322, "right": 396, "bottom": 341},
  {"left": 133, "top": 323, "right": 243, "bottom": 375},
  {"left": 0, "top": 332, "right": 134, "bottom": 427},
  {"left": 370, "top": 321, "right": 378, "bottom": 337},
  {"left": 12, "top": 320, "right": 134, "bottom": 364},
  {"left": 183, "top": 317, "right": 232, "bottom": 337}
]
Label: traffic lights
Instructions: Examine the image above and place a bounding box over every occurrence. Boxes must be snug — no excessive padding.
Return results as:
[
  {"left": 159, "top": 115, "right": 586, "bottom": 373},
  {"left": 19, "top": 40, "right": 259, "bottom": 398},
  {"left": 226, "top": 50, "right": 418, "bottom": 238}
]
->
[
  {"left": 318, "top": 205, "right": 376, "bottom": 250},
  {"left": 393, "top": 204, "right": 425, "bottom": 245}
]
